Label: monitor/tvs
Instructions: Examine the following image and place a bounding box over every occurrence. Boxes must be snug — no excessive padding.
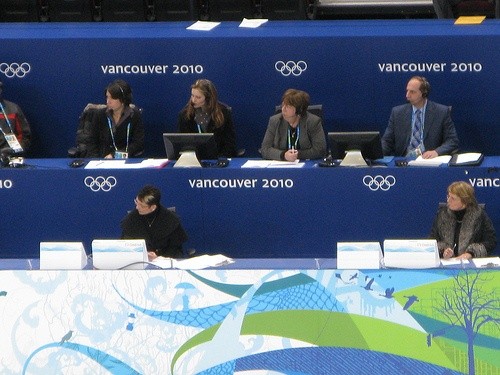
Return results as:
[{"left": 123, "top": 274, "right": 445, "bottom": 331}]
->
[
  {"left": 163, "top": 133, "right": 219, "bottom": 167},
  {"left": 328, "top": 131, "right": 384, "bottom": 166}
]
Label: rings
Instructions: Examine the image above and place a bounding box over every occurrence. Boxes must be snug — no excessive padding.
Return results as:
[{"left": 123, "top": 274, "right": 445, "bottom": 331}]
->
[{"left": 465, "top": 255, "right": 467, "bottom": 257}]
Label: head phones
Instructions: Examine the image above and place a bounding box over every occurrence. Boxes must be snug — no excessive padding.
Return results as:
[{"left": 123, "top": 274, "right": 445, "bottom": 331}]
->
[
  {"left": 119, "top": 86, "right": 131, "bottom": 104},
  {"left": 423, "top": 78, "right": 428, "bottom": 97},
  {"left": 297, "top": 99, "right": 308, "bottom": 115}
]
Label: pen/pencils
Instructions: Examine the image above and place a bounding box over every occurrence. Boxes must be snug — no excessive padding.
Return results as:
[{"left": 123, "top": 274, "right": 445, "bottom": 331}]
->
[
  {"left": 292, "top": 146, "right": 294, "bottom": 154},
  {"left": 452, "top": 243, "right": 456, "bottom": 250}
]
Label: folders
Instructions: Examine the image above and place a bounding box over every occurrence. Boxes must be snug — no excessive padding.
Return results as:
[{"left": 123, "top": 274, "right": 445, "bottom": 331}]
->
[
  {"left": 446, "top": 152, "right": 486, "bottom": 167},
  {"left": 440, "top": 255, "right": 500, "bottom": 271}
]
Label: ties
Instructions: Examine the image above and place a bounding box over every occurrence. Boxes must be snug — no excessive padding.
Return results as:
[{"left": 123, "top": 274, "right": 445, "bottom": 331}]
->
[{"left": 410, "top": 110, "right": 422, "bottom": 150}]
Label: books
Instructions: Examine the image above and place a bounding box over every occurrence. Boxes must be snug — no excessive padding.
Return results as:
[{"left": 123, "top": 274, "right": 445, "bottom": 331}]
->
[{"left": 449, "top": 150, "right": 484, "bottom": 166}]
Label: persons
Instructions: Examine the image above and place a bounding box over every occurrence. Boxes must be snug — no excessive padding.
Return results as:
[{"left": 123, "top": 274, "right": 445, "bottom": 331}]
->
[
  {"left": 0, "top": 80, "right": 32, "bottom": 166},
  {"left": 86, "top": 77, "right": 147, "bottom": 161},
  {"left": 116, "top": 185, "right": 185, "bottom": 262},
  {"left": 122, "top": 154, "right": 127, "bottom": 159},
  {"left": 181, "top": 77, "right": 238, "bottom": 159},
  {"left": 260, "top": 88, "right": 327, "bottom": 161},
  {"left": 12, "top": 136, "right": 16, "bottom": 142},
  {"left": 380, "top": 74, "right": 460, "bottom": 159},
  {"left": 431, "top": 178, "right": 498, "bottom": 262}
]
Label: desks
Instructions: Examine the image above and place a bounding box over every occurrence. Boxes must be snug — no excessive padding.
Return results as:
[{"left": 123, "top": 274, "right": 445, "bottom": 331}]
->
[
  {"left": 0, "top": 258, "right": 500, "bottom": 375},
  {"left": 0, "top": 19, "right": 500, "bottom": 159},
  {"left": 0, "top": 157, "right": 500, "bottom": 259}
]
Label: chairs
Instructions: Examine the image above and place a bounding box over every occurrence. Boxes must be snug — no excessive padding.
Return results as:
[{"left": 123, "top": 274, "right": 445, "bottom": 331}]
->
[{"left": 76, "top": 103, "right": 143, "bottom": 158}]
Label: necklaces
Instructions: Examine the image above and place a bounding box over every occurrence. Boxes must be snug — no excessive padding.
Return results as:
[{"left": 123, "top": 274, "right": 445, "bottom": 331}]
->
[
  {"left": 144, "top": 213, "right": 158, "bottom": 228},
  {"left": 113, "top": 110, "right": 122, "bottom": 119}
]
